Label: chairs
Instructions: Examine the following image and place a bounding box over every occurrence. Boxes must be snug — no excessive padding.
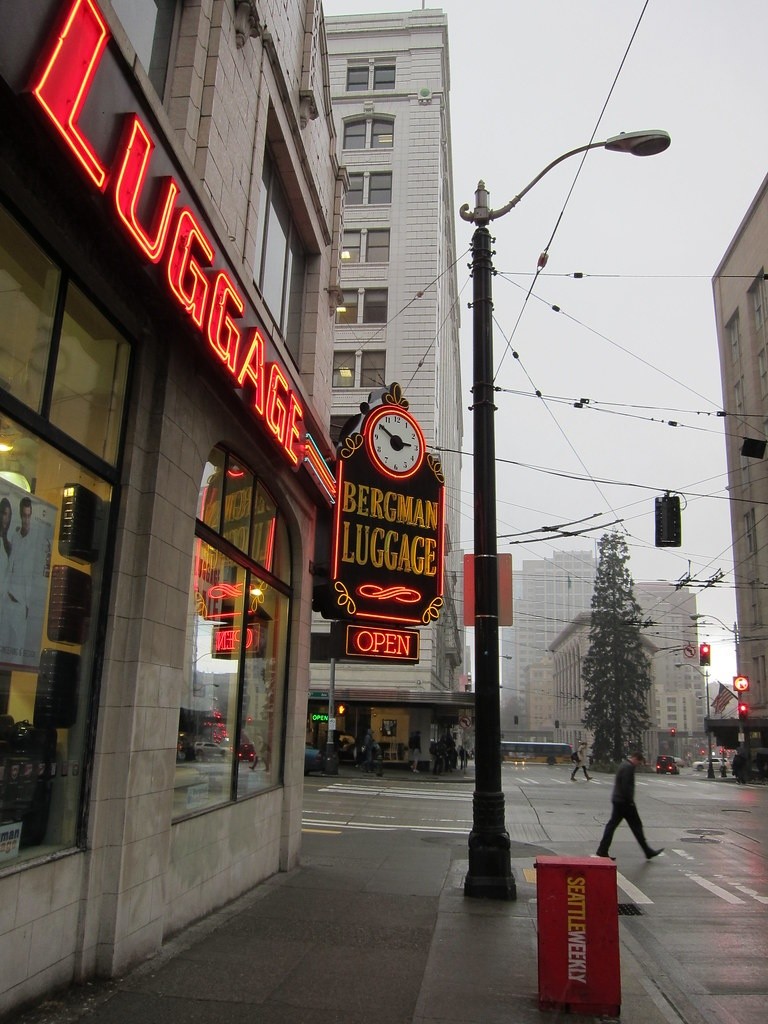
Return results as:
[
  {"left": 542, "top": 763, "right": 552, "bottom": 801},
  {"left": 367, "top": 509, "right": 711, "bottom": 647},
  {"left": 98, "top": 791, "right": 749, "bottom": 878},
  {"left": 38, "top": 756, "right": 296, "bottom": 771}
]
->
[{"left": 383, "top": 741, "right": 399, "bottom": 761}]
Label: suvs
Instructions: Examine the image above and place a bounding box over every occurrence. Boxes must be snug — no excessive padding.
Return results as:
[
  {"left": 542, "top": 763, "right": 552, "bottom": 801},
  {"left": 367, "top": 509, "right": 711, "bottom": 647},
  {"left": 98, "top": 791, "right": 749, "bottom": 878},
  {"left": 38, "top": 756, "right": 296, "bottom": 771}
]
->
[{"left": 693, "top": 757, "right": 731, "bottom": 772}]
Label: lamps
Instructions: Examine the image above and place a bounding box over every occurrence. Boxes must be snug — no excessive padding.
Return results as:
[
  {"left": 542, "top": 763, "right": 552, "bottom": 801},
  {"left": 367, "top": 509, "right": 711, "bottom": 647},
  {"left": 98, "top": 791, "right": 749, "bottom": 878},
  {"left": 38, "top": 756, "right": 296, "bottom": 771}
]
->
[{"left": 0, "top": 459, "right": 31, "bottom": 492}]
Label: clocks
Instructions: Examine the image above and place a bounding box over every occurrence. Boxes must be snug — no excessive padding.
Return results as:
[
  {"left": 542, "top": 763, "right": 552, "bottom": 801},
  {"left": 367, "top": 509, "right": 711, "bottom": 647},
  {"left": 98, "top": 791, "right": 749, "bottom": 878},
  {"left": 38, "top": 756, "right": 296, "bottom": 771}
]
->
[{"left": 369, "top": 410, "right": 421, "bottom": 474}]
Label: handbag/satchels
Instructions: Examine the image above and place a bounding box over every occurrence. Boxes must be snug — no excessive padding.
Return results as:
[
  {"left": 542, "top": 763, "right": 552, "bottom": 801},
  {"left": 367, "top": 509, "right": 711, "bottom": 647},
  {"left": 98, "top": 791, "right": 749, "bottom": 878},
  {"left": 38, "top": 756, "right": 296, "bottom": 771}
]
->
[{"left": 571, "top": 750, "right": 580, "bottom": 763}]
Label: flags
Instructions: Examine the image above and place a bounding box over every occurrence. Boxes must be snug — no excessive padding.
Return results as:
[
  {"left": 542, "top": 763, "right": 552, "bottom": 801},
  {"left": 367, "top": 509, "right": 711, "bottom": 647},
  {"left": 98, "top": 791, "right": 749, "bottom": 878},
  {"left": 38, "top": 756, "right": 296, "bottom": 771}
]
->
[{"left": 711, "top": 684, "right": 733, "bottom": 713}]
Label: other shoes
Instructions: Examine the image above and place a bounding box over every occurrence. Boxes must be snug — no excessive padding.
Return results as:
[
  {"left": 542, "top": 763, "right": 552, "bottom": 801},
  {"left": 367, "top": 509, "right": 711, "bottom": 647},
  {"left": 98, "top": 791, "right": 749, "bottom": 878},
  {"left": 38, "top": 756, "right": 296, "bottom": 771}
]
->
[
  {"left": 413, "top": 769, "right": 419, "bottom": 773},
  {"left": 571, "top": 778, "right": 577, "bottom": 781},
  {"left": 588, "top": 777, "right": 593, "bottom": 780},
  {"left": 647, "top": 847, "right": 666, "bottom": 858},
  {"left": 597, "top": 852, "right": 617, "bottom": 860}
]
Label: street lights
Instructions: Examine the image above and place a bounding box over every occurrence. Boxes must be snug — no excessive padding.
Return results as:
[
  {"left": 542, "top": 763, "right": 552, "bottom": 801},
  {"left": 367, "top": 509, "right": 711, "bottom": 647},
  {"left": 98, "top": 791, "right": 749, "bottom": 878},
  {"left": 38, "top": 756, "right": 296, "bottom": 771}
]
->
[
  {"left": 674, "top": 662, "right": 717, "bottom": 779},
  {"left": 460, "top": 128, "right": 671, "bottom": 899},
  {"left": 689, "top": 613, "right": 743, "bottom": 719}
]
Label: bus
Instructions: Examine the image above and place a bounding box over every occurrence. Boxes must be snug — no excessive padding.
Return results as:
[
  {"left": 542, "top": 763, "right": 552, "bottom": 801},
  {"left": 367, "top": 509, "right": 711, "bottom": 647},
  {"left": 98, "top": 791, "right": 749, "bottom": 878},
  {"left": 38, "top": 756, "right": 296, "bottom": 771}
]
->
[{"left": 500, "top": 740, "right": 573, "bottom": 766}]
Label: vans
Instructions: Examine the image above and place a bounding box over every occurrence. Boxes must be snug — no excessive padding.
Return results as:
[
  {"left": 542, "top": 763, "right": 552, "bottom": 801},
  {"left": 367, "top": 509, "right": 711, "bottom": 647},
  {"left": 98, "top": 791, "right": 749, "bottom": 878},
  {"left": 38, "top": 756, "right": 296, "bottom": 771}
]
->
[{"left": 656, "top": 755, "right": 679, "bottom": 775}]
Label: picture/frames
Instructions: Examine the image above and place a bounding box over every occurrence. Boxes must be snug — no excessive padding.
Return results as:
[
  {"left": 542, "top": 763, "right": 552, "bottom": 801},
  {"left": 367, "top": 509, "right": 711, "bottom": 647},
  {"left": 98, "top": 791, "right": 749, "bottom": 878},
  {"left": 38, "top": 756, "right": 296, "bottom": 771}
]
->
[{"left": 381, "top": 719, "right": 397, "bottom": 737}]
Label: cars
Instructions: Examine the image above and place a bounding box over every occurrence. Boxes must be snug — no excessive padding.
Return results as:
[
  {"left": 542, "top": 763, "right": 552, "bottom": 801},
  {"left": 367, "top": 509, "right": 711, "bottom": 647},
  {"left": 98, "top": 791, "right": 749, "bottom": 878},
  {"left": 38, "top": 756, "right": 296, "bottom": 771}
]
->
[
  {"left": 674, "top": 757, "right": 686, "bottom": 768},
  {"left": 176, "top": 735, "right": 254, "bottom": 763},
  {"left": 304, "top": 743, "right": 326, "bottom": 776}
]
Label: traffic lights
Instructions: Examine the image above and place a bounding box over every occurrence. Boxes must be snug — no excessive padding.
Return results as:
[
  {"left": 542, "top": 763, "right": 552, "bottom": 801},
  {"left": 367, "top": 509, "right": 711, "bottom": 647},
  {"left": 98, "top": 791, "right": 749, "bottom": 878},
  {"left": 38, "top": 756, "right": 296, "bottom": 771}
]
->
[
  {"left": 740, "top": 703, "right": 748, "bottom": 719},
  {"left": 338, "top": 704, "right": 346, "bottom": 714},
  {"left": 671, "top": 728, "right": 675, "bottom": 734},
  {"left": 734, "top": 676, "right": 749, "bottom": 692},
  {"left": 700, "top": 644, "right": 710, "bottom": 666}
]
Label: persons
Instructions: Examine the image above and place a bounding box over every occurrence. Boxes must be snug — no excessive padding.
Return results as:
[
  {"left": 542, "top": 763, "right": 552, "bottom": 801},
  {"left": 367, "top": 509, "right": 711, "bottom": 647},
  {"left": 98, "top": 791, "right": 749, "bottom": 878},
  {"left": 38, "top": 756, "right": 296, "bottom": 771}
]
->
[
  {"left": 432, "top": 734, "right": 458, "bottom": 775},
  {"left": 0, "top": 497, "right": 14, "bottom": 663},
  {"left": 570, "top": 740, "right": 593, "bottom": 782},
  {"left": 6, "top": 497, "right": 35, "bottom": 664},
  {"left": 731, "top": 754, "right": 746, "bottom": 785},
  {"left": 459, "top": 746, "right": 468, "bottom": 770},
  {"left": 248, "top": 730, "right": 269, "bottom": 772},
  {"left": 0, "top": 713, "right": 57, "bottom": 849},
  {"left": 756, "top": 752, "right": 767, "bottom": 785},
  {"left": 596, "top": 750, "right": 666, "bottom": 861},
  {"left": 409, "top": 731, "right": 423, "bottom": 774},
  {"left": 355, "top": 728, "right": 376, "bottom": 774}
]
